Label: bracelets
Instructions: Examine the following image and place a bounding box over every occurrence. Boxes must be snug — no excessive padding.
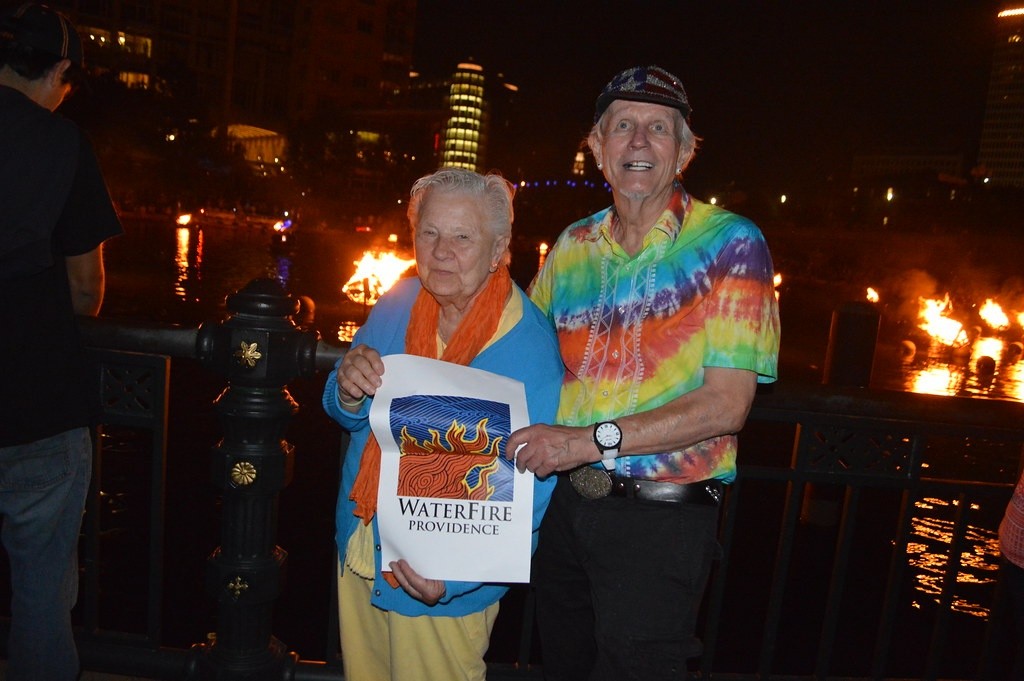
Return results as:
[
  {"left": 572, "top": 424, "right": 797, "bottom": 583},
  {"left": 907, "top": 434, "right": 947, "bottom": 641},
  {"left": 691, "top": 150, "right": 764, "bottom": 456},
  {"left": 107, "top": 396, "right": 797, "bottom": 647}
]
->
[{"left": 337, "top": 392, "right": 367, "bottom": 407}]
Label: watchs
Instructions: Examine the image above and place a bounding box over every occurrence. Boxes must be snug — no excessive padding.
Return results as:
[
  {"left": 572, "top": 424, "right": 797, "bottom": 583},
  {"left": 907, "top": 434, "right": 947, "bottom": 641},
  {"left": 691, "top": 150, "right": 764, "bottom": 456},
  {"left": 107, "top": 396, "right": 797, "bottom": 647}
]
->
[{"left": 593, "top": 421, "right": 621, "bottom": 470}]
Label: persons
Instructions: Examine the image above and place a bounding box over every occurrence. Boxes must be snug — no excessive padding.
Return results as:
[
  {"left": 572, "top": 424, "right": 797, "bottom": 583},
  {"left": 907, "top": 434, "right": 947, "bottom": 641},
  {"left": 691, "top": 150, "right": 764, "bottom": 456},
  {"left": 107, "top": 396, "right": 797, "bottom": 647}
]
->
[
  {"left": 322, "top": 168, "right": 568, "bottom": 681},
  {"left": 995, "top": 467, "right": 1024, "bottom": 681},
  {"left": 504, "top": 62, "right": 782, "bottom": 681},
  {"left": 0, "top": 3, "right": 123, "bottom": 681}
]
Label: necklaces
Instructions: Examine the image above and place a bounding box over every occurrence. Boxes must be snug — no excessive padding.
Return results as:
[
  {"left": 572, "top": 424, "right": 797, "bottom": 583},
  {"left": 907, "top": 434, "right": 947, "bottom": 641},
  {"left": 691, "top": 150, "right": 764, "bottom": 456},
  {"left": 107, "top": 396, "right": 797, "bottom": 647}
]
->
[{"left": 439, "top": 327, "right": 446, "bottom": 340}]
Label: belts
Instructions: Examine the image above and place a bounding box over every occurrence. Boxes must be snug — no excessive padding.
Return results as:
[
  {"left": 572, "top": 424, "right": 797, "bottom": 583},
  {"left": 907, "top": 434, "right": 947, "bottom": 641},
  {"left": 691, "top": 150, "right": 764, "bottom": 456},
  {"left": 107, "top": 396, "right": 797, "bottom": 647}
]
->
[{"left": 559, "top": 465, "right": 721, "bottom": 507}]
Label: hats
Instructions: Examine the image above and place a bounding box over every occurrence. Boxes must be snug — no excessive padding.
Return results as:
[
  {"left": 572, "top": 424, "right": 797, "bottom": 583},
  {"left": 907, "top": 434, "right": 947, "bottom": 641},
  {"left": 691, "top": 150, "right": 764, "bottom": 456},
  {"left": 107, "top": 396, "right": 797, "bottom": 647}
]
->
[
  {"left": 593, "top": 66, "right": 690, "bottom": 121},
  {"left": 0, "top": 0, "right": 90, "bottom": 77}
]
